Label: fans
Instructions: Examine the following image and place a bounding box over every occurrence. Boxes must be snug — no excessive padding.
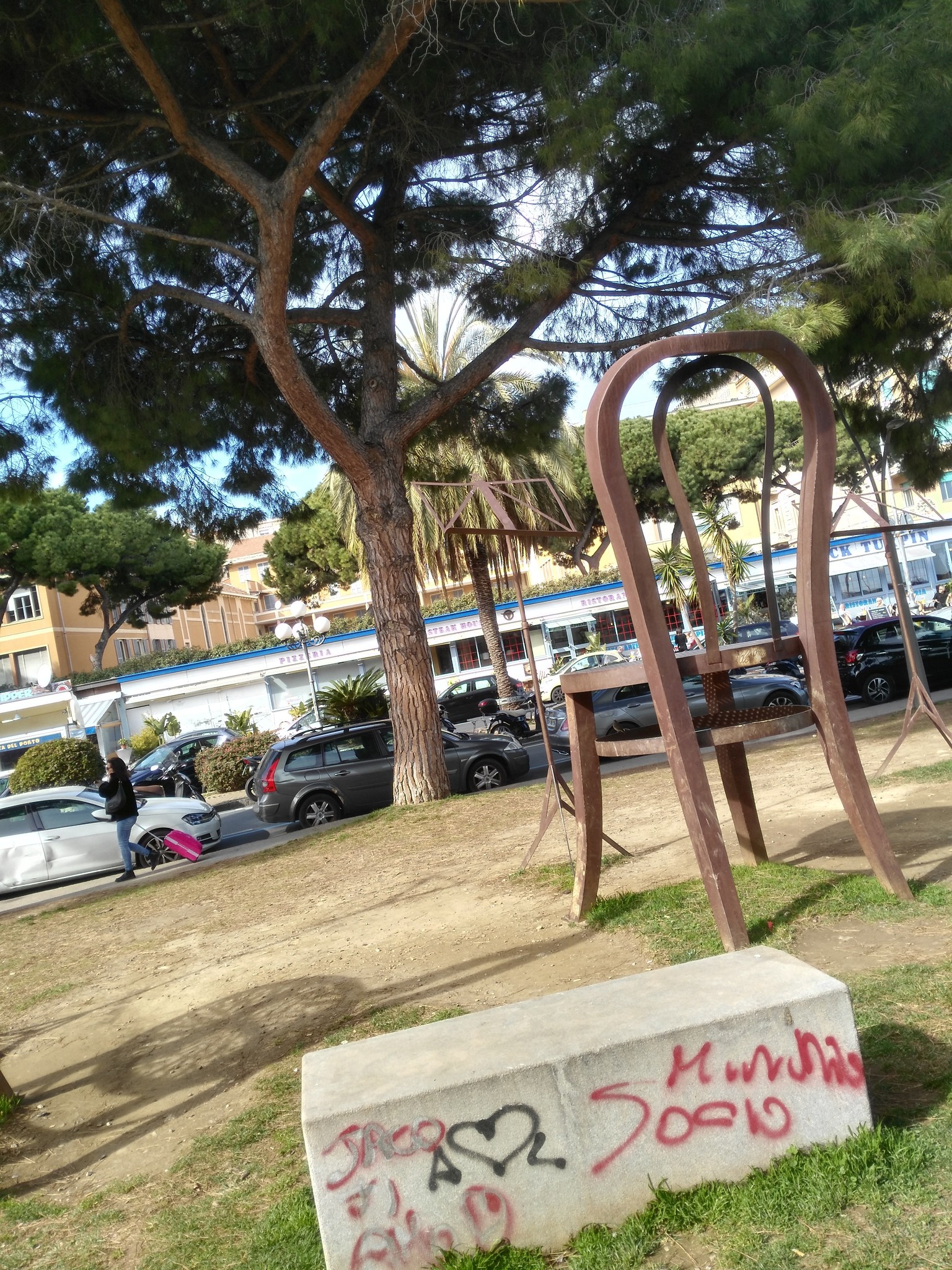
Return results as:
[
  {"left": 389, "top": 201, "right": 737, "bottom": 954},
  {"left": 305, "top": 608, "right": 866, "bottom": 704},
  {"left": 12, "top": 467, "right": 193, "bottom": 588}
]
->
[{"left": 2, "top": 710, "right": 32, "bottom": 723}]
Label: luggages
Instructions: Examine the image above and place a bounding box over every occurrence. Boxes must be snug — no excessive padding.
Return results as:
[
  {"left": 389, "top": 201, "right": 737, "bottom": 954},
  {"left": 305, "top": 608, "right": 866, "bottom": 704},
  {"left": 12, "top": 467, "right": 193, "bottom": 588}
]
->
[{"left": 134, "top": 822, "right": 202, "bottom": 863}]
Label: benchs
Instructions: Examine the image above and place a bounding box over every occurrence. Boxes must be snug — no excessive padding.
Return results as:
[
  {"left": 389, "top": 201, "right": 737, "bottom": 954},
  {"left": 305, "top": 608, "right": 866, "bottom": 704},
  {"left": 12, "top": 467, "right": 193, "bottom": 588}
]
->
[{"left": 467, "top": 695, "right": 534, "bottom": 733}]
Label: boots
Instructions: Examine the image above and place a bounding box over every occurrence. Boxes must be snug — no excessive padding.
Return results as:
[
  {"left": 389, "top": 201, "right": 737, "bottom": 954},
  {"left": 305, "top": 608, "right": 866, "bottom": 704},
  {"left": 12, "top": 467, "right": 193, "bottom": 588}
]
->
[
  {"left": 115, "top": 869, "right": 136, "bottom": 882},
  {"left": 147, "top": 850, "right": 160, "bottom": 871}
]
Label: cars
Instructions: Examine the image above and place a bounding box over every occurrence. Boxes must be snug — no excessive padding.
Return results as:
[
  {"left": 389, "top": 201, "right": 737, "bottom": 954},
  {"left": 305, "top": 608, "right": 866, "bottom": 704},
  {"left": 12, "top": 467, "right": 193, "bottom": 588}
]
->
[
  {"left": 433, "top": 673, "right": 530, "bottom": 722},
  {"left": 539, "top": 671, "right": 812, "bottom": 762},
  {"left": 128, "top": 727, "right": 248, "bottom": 770},
  {"left": 835, "top": 615, "right": 951, "bottom": 703},
  {"left": 126, "top": 735, "right": 219, "bottom": 798},
  {"left": 0, "top": 785, "right": 221, "bottom": 892},
  {"left": 531, "top": 649, "right": 629, "bottom": 704},
  {"left": 682, "top": 620, "right": 802, "bottom": 668},
  {"left": 0, "top": 775, "right": 19, "bottom": 797}
]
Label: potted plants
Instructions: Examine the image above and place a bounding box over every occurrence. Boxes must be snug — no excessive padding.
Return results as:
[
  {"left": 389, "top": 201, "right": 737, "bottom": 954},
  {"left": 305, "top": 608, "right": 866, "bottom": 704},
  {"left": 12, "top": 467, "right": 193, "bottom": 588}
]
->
[{"left": 118, "top": 738, "right": 128, "bottom": 749}]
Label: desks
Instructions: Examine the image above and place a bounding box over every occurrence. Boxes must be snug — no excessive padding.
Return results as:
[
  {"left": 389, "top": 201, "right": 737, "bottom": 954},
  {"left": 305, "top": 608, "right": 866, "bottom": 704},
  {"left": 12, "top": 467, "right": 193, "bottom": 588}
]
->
[{"left": 922, "top": 601, "right": 935, "bottom": 609}]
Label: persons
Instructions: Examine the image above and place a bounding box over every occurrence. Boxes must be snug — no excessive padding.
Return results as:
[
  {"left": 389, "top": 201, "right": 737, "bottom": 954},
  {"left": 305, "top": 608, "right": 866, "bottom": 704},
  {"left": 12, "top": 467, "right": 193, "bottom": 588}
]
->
[
  {"left": 675, "top": 627, "right": 688, "bottom": 652},
  {"left": 838, "top": 604, "right": 861, "bottom": 626},
  {"left": 876, "top": 598, "right": 886, "bottom": 608},
  {"left": 932, "top": 586, "right": 952, "bottom": 610},
  {"left": 99, "top": 757, "right": 161, "bottom": 883},
  {"left": 905, "top": 589, "right": 916, "bottom": 604},
  {"left": 684, "top": 632, "right": 699, "bottom": 649},
  {"left": 617, "top": 646, "right": 630, "bottom": 658}
]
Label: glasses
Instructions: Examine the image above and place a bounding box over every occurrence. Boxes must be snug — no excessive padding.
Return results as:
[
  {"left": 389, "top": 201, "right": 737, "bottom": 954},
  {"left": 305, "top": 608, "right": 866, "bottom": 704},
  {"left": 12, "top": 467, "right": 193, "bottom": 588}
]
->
[
  {"left": 106, "top": 767, "right": 113, "bottom": 771},
  {"left": 939, "top": 589, "right": 943, "bottom": 590}
]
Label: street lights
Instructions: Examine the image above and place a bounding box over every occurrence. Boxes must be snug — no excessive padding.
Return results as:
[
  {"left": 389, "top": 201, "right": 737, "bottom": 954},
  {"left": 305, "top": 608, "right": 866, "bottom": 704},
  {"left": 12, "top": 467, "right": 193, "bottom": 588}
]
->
[{"left": 272, "top": 601, "right": 330, "bottom": 724}]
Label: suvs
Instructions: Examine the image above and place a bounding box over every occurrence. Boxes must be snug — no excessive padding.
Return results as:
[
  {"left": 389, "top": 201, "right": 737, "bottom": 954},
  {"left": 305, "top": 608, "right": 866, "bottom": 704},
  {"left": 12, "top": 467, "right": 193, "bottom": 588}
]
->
[{"left": 252, "top": 718, "right": 531, "bottom": 831}]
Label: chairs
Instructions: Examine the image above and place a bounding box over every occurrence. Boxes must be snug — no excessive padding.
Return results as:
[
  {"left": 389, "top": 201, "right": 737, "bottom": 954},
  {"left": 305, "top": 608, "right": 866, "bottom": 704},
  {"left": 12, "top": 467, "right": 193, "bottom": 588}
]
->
[
  {"left": 189, "top": 747, "right": 196, "bottom": 758},
  {"left": 916, "top": 602, "right": 932, "bottom": 616},
  {"left": 944, "top": 596, "right": 952, "bottom": 604},
  {"left": 844, "top": 615, "right": 852, "bottom": 624},
  {"left": 560, "top": 330, "right": 915, "bottom": 951},
  {"left": 886, "top": 626, "right": 900, "bottom": 643}
]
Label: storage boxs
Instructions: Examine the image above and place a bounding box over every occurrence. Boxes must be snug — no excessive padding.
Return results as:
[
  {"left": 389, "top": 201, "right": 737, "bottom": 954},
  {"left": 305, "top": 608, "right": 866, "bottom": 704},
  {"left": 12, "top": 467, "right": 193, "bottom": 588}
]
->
[{"left": 478, "top": 698, "right": 498, "bottom": 714}]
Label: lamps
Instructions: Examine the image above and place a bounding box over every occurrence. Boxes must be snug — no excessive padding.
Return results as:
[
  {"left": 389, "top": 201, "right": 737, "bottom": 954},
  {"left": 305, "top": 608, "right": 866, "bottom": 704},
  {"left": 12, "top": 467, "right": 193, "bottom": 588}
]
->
[{"left": 62, "top": 709, "right": 67, "bottom": 714}]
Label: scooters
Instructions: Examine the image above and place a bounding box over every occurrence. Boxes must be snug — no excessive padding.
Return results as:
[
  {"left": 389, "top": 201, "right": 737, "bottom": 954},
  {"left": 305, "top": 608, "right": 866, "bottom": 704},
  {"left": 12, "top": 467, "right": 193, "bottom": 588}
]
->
[
  {"left": 436, "top": 688, "right": 547, "bottom": 745},
  {"left": 149, "top": 749, "right": 207, "bottom": 802},
  {"left": 233, "top": 755, "right": 263, "bottom": 802}
]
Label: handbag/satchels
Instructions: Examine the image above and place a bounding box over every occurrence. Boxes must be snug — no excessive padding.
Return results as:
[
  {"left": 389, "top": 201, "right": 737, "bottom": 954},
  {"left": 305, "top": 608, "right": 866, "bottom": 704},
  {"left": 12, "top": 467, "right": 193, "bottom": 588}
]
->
[{"left": 105, "top": 781, "right": 126, "bottom": 812}]
